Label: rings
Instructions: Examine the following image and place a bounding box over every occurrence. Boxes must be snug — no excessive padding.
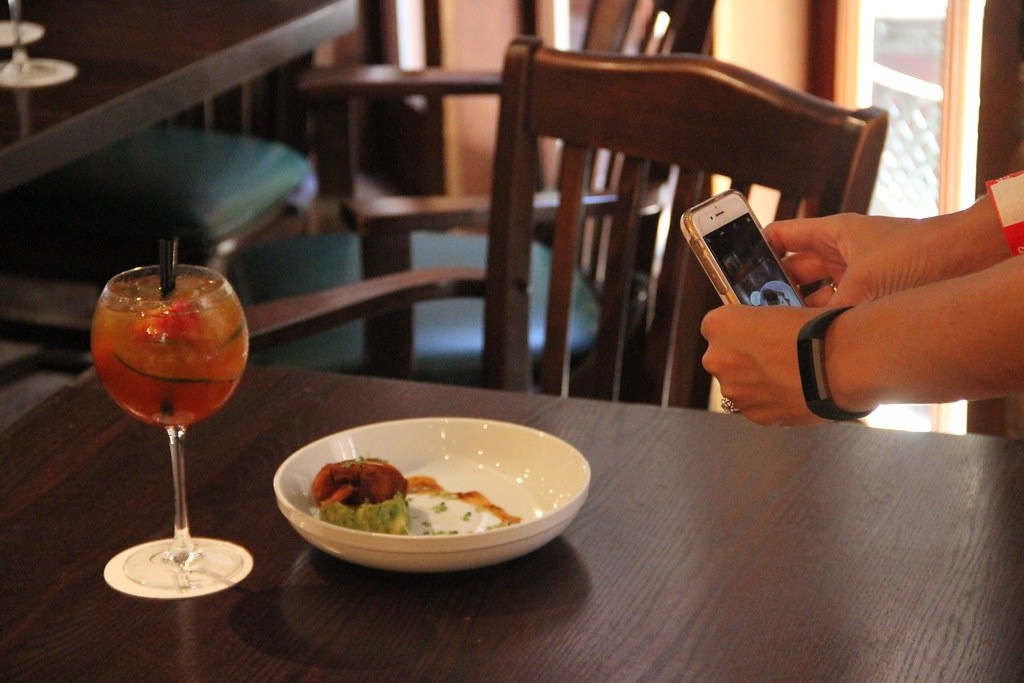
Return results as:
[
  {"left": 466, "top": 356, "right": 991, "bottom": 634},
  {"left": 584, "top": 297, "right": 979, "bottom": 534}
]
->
[
  {"left": 719, "top": 398, "right": 740, "bottom": 414},
  {"left": 832, "top": 284, "right": 843, "bottom": 296}
]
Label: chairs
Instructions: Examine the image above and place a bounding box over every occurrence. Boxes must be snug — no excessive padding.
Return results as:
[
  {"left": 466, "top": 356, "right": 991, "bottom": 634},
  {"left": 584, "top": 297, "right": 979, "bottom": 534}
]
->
[
  {"left": 0, "top": 127, "right": 318, "bottom": 354},
  {"left": 242, "top": 35, "right": 890, "bottom": 413},
  {"left": 226, "top": 0, "right": 715, "bottom": 379}
]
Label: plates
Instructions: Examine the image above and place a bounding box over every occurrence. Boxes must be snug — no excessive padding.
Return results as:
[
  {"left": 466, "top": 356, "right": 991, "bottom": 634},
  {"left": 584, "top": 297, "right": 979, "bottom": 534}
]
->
[{"left": 273, "top": 416, "right": 591, "bottom": 572}]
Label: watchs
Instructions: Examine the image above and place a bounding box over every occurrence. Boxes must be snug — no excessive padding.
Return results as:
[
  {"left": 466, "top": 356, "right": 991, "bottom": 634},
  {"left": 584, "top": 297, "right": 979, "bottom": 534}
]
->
[{"left": 796, "top": 304, "right": 877, "bottom": 419}]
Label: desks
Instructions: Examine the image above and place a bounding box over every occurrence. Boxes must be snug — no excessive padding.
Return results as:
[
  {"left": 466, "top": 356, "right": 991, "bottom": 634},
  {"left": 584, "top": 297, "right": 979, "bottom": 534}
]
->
[
  {"left": 0, "top": 0, "right": 360, "bottom": 200},
  {"left": 0, "top": 365, "right": 1024, "bottom": 683}
]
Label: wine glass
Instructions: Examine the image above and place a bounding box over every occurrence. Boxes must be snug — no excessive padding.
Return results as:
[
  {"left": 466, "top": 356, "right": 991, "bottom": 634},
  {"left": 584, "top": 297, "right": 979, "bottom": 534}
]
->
[
  {"left": 91, "top": 264, "right": 251, "bottom": 590},
  {"left": 0, "top": 0, "right": 79, "bottom": 88}
]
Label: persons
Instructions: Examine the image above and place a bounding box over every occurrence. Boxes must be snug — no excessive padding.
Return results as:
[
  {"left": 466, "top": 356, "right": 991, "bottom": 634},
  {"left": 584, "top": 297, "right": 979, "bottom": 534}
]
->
[{"left": 699, "top": 170, "right": 1023, "bottom": 427}]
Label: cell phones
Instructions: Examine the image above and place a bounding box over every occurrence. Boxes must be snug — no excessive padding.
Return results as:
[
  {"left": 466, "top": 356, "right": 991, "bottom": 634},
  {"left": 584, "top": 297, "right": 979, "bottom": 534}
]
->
[{"left": 679, "top": 189, "right": 808, "bottom": 310}]
